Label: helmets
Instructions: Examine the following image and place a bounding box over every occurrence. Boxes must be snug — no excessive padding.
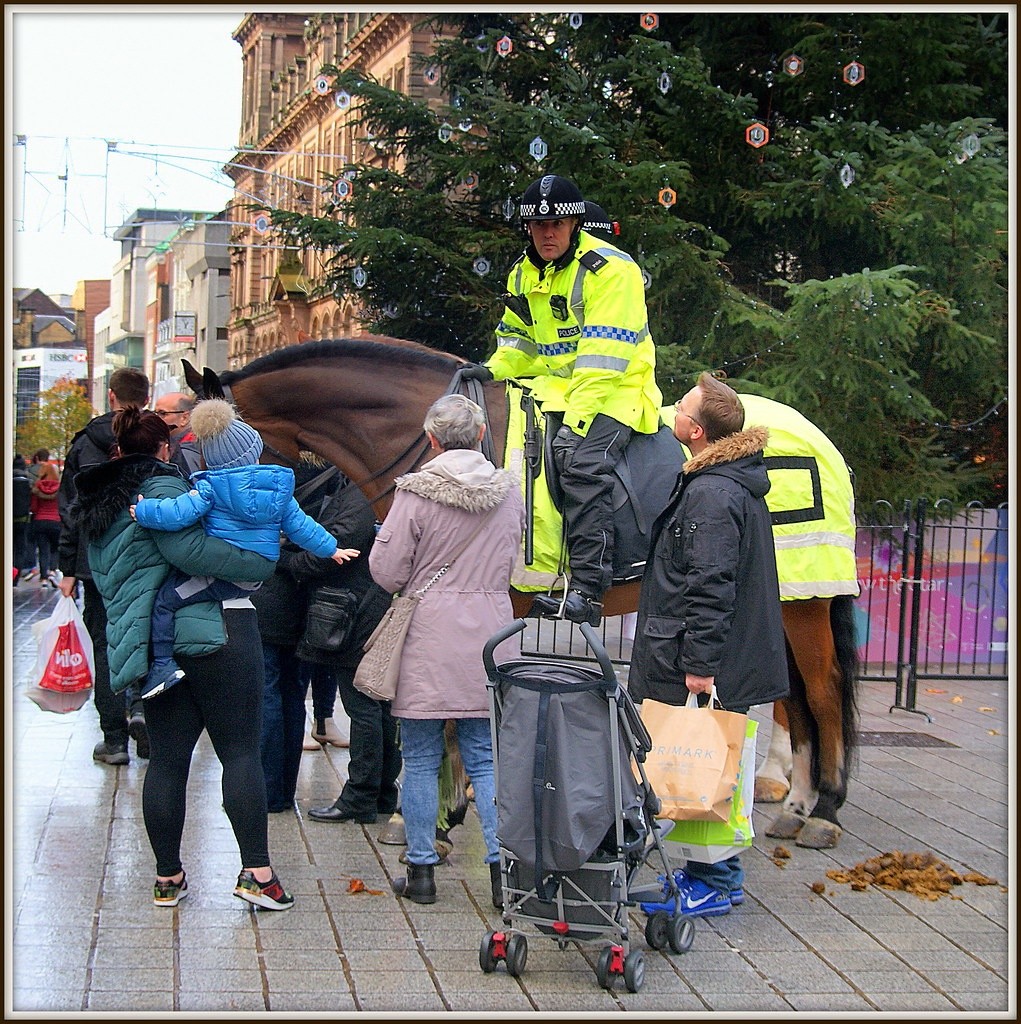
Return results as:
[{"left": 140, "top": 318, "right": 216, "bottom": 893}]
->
[
  {"left": 520, "top": 175, "right": 586, "bottom": 221},
  {"left": 580, "top": 202, "right": 613, "bottom": 243}
]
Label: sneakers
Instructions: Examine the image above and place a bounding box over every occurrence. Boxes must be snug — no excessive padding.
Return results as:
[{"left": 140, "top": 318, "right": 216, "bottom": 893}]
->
[
  {"left": 140, "top": 659, "right": 185, "bottom": 699},
  {"left": 92, "top": 739, "right": 130, "bottom": 763},
  {"left": 658, "top": 867, "right": 746, "bottom": 904},
  {"left": 128, "top": 713, "right": 152, "bottom": 759},
  {"left": 234, "top": 866, "right": 295, "bottom": 909},
  {"left": 152, "top": 870, "right": 188, "bottom": 907},
  {"left": 639, "top": 878, "right": 733, "bottom": 920}
]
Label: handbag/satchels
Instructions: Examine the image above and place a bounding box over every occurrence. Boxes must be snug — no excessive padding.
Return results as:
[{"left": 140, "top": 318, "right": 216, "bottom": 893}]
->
[
  {"left": 299, "top": 588, "right": 357, "bottom": 653},
  {"left": 351, "top": 597, "right": 416, "bottom": 700},
  {"left": 634, "top": 684, "right": 759, "bottom": 863},
  {"left": 25, "top": 595, "right": 95, "bottom": 713}
]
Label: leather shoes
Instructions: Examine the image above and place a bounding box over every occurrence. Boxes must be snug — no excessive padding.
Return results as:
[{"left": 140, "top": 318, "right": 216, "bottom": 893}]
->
[
  {"left": 307, "top": 803, "right": 375, "bottom": 824},
  {"left": 531, "top": 591, "right": 602, "bottom": 628}
]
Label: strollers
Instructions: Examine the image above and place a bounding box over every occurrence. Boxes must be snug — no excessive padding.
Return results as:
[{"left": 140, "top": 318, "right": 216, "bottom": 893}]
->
[{"left": 476, "top": 616, "right": 698, "bottom": 996}]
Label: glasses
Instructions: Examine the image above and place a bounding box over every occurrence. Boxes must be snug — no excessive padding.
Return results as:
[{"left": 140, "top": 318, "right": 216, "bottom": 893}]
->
[
  {"left": 674, "top": 400, "right": 705, "bottom": 434},
  {"left": 151, "top": 410, "right": 187, "bottom": 419}
]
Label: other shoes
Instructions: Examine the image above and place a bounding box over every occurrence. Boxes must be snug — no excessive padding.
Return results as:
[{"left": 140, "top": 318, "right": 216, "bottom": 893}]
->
[{"left": 24, "top": 567, "right": 59, "bottom": 587}]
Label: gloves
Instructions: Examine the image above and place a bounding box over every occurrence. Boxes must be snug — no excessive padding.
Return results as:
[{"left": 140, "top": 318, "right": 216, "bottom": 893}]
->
[
  {"left": 554, "top": 425, "right": 587, "bottom": 472},
  {"left": 457, "top": 362, "right": 493, "bottom": 387}
]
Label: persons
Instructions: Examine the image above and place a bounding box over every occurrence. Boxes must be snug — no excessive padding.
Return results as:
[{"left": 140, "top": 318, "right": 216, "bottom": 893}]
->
[
  {"left": 73, "top": 408, "right": 296, "bottom": 910},
  {"left": 223, "top": 453, "right": 399, "bottom": 824},
  {"left": 12, "top": 448, "right": 62, "bottom": 589},
  {"left": 625, "top": 373, "right": 789, "bottom": 917},
  {"left": 58, "top": 367, "right": 193, "bottom": 764},
  {"left": 576, "top": 200, "right": 619, "bottom": 246},
  {"left": 368, "top": 394, "right": 527, "bottom": 907},
  {"left": 461, "top": 176, "right": 663, "bottom": 627},
  {"left": 131, "top": 400, "right": 360, "bottom": 701},
  {"left": 153, "top": 392, "right": 202, "bottom": 477}
]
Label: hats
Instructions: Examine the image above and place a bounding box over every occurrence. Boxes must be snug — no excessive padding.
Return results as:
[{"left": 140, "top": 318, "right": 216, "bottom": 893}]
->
[{"left": 189, "top": 397, "right": 264, "bottom": 472}]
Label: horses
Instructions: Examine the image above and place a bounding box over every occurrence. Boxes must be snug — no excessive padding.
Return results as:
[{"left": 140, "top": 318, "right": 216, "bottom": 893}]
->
[{"left": 181, "top": 336, "right": 861, "bottom": 850}]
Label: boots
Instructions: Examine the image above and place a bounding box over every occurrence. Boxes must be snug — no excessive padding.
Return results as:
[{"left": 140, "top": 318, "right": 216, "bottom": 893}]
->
[
  {"left": 490, "top": 861, "right": 506, "bottom": 907},
  {"left": 312, "top": 717, "right": 350, "bottom": 747},
  {"left": 392, "top": 862, "right": 437, "bottom": 902},
  {"left": 302, "top": 732, "right": 321, "bottom": 749}
]
